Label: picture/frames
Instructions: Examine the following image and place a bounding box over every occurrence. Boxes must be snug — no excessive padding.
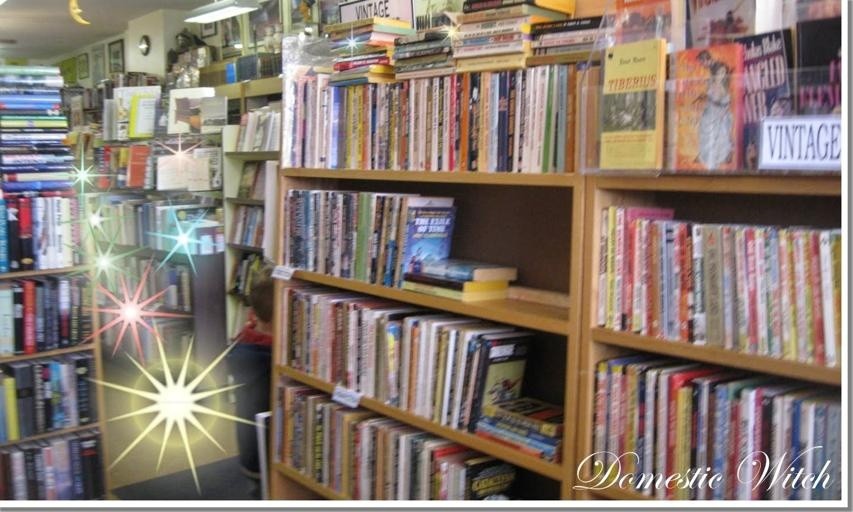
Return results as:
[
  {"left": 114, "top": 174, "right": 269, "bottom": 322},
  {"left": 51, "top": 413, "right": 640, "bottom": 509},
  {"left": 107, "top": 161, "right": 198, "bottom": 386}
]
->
[
  {"left": 199, "top": 22, "right": 217, "bottom": 39},
  {"left": 108, "top": 39, "right": 124, "bottom": 73}
]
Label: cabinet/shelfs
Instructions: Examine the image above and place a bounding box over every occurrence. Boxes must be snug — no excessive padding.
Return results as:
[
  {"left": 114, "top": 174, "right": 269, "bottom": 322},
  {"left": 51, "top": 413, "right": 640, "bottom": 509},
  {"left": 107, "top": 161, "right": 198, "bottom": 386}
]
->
[{"left": 0, "top": 48, "right": 842, "bottom": 501}]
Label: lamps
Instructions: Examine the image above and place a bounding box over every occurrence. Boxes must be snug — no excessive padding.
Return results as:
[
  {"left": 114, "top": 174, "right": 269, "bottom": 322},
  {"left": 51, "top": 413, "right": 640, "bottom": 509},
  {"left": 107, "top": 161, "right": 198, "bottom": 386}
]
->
[{"left": 183, "top": 0, "right": 262, "bottom": 25}]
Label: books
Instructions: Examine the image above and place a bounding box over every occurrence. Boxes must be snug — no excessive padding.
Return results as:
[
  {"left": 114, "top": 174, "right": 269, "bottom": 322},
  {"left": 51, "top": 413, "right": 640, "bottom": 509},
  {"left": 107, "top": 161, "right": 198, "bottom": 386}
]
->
[{"left": 0, "top": 1, "right": 843, "bottom": 501}]
